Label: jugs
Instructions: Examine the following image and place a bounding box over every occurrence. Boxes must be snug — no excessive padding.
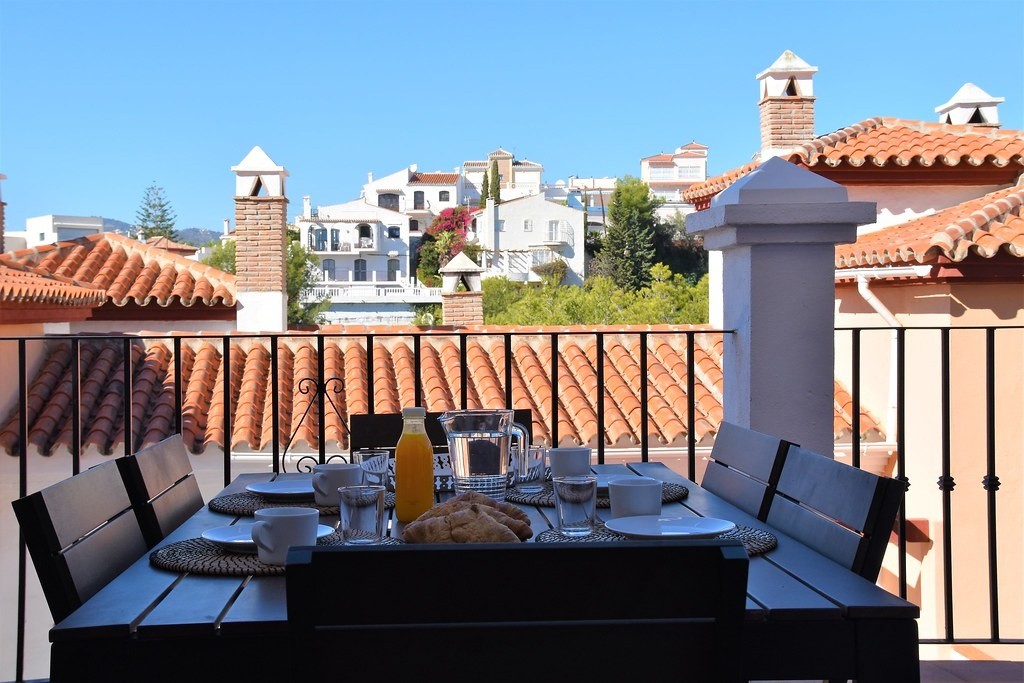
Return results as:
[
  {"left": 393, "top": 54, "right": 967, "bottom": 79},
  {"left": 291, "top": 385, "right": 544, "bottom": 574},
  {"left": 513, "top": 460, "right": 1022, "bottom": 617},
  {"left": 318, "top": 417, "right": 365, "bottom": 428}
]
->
[{"left": 436, "top": 408, "right": 530, "bottom": 504}]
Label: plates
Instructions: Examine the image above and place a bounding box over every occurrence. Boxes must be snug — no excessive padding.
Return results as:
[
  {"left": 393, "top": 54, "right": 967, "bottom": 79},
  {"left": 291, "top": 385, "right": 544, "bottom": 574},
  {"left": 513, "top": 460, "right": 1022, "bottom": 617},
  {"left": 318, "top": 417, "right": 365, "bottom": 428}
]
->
[
  {"left": 591, "top": 473, "right": 660, "bottom": 493},
  {"left": 246, "top": 480, "right": 316, "bottom": 502},
  {"left": 201, "top": 523, "right": 335, "bottom": 554},
  {"left": 605, "top": 514, "right": 736, "bottom": 540}
]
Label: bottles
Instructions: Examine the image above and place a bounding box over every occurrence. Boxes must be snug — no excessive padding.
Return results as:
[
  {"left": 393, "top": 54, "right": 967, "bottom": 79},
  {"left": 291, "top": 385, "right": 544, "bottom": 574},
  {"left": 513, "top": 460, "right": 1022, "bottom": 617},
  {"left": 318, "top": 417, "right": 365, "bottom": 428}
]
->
[{"left": 394, "top": 407, "right": 434, "bottom": 523}]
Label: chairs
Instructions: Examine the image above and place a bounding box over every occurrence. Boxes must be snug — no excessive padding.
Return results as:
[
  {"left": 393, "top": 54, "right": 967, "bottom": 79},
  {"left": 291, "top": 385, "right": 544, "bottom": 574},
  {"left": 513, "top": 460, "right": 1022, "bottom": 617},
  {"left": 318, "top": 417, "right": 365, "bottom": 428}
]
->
[
  {"left": 701, "top": 421, "right": 800, "bottom": 523},
  {"left": 350, "top": 408, "right": 532, "bottom": 464},
  {"left": 766, "top": 444, "right": 907, "bottom": 585},
  {"left": 11, "top": 459, "right": 149, "bottom": 626},
  {"left": 286, "top": 539, "right": 751, "bottom": 682},
  {"left": 89, "top": 433, "right": 205, "bottom": 551}
]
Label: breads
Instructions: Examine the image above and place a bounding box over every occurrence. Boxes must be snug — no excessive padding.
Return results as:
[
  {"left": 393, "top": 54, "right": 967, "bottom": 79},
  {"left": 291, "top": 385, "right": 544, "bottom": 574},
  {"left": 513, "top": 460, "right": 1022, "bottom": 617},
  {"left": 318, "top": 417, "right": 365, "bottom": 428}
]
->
[{"left": 402, "top": 490, "right": 534, "bottom": 544}]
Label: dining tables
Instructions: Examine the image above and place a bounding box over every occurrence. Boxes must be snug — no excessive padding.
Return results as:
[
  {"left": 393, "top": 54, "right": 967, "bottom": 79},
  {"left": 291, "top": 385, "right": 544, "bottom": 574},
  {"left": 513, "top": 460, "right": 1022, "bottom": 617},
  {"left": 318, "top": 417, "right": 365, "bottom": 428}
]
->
[{"left": 49, "top": 462, "right": 920, "bottom": 683}]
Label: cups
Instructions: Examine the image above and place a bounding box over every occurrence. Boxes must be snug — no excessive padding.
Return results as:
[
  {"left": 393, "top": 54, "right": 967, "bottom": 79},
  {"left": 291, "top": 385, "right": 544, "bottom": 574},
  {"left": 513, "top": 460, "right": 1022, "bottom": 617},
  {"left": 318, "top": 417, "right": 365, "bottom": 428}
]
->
[
  {"left": 552, "top": 476, "right": 597, "bottom": 537},
  {"left": 337, "top": 485, "right": 385, "bottom": 546},
  {"left": 608, "top": 478, "right": 662, "bottom": 518},
  {"left": 549, "top": 447, "right": 591, "bottom": 480},
  {"left": 511, "top": 446, "right": 546, "bottom": 493},
  {"left": 251, "top": 506, "right": 320, "bottom": 566},
  {"left": 312, "top": 463, "right": 361, "bottom": 506},
  {"left": 353, "top": 450, "right": 389, "bottom": 491}
]
